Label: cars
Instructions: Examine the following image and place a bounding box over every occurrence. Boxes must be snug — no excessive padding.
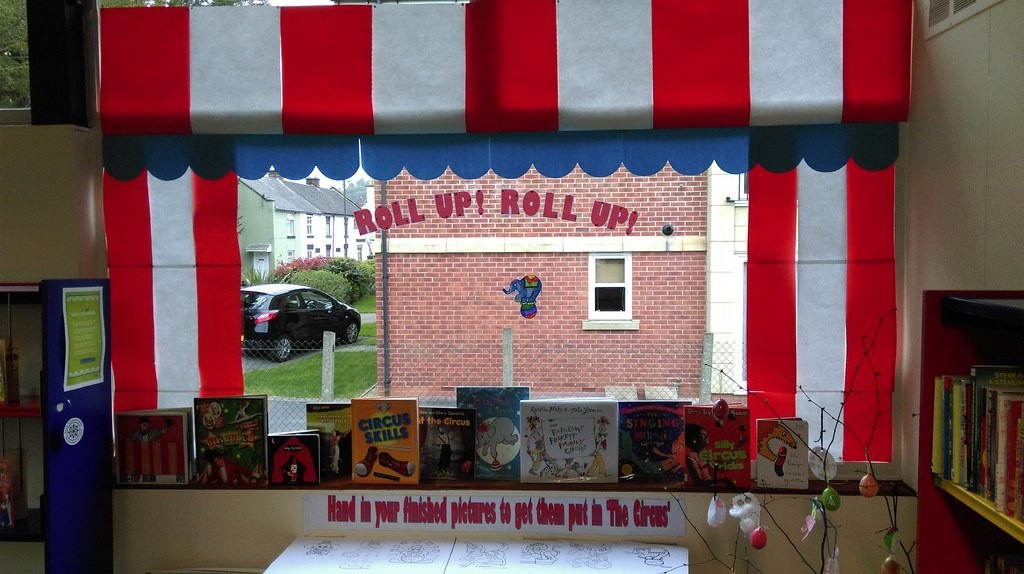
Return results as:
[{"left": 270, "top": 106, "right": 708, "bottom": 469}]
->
[{"left": 240, "top": 284, "right": 361, "bottom": 363}]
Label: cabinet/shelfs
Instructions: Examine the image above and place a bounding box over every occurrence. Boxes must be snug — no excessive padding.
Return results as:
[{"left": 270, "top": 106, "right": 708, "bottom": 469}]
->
[{"left": 936, "top": 297, "right": 1024, "bottom": 544}]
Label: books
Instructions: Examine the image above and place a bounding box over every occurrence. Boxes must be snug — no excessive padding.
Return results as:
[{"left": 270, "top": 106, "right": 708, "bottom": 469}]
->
[
  {"left": 193, "top": 395, "right": 321, "bottom": 490},
  {"left": 306, "top": 387, "right": 751, "bottom": 489},
  {"left": 931, "top": 364, "right": 1024, "bottom": 522},
  {"left": 117, "top": 408, "right": 194, "bottom": 486},
  {"left": 756, "top": 418, "right": 809, "bottom": 491}
]
